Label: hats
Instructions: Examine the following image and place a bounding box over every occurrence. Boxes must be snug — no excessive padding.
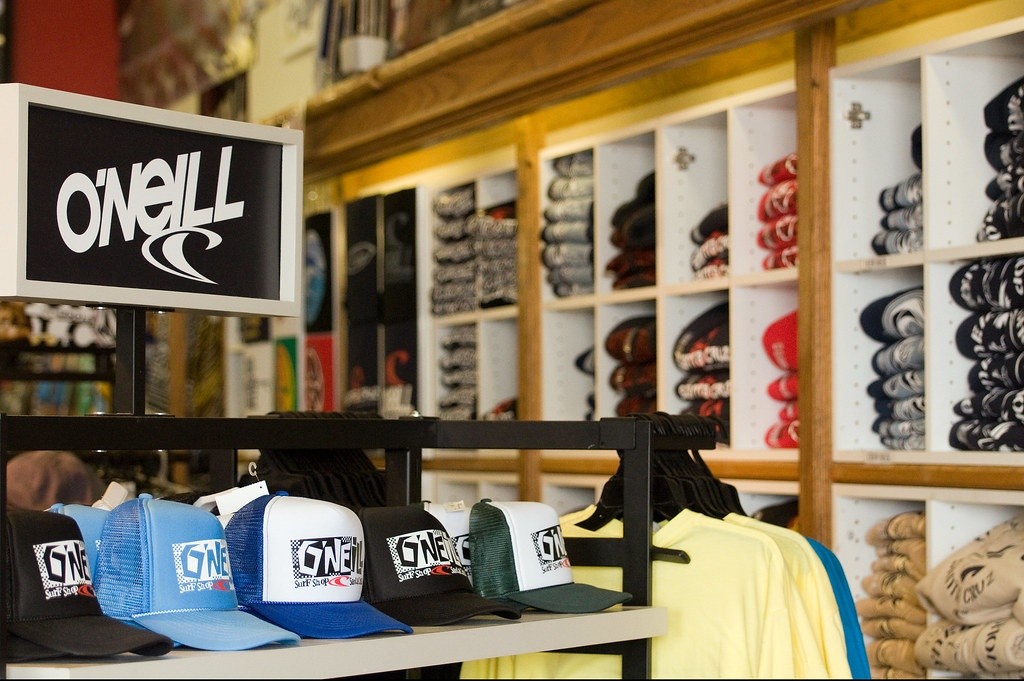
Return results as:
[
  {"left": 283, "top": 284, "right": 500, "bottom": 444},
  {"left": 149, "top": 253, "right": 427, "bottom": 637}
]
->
[
  {"left": 224, "top": 490, "right": 413, "bottom": 639},
  {"left": 468, "top": 498, "right": 633, "bottom": 613},
  {"left": 45, "top": 504, "right": 110, "bottom": 584},
  {"left": 0, "top": 508, "right": 174, "bottom": 661},
  {"left": 356, "top": 505, "right": 521, "bottom": 626},
  {"left": 94, "top": 492, "right": 301, "bottom": 652},
  {"left": 429, "top": 508, "right": 473, "bottom": 585}
]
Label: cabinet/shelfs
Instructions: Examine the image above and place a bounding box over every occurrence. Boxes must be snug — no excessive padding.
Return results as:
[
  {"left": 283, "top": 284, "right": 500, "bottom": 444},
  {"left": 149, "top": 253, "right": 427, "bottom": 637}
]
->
[
  {"left": 0, "top": 409, "right": 667, "bottom": 680},
  {"left": 291, "top": 0, "right": 1024, "bottom": 678}
]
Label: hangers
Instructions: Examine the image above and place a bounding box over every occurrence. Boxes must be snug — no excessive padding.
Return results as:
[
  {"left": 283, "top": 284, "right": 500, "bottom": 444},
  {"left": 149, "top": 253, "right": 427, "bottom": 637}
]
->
[
  {"left": 577, "top": 413, "right": 747, "bottom": 531},
  {"left": 134, "top": 447, "right": 191, "bottom": 494},
  {"left": 235, "top": 409, "right": 389, "bottom": 509}
]
